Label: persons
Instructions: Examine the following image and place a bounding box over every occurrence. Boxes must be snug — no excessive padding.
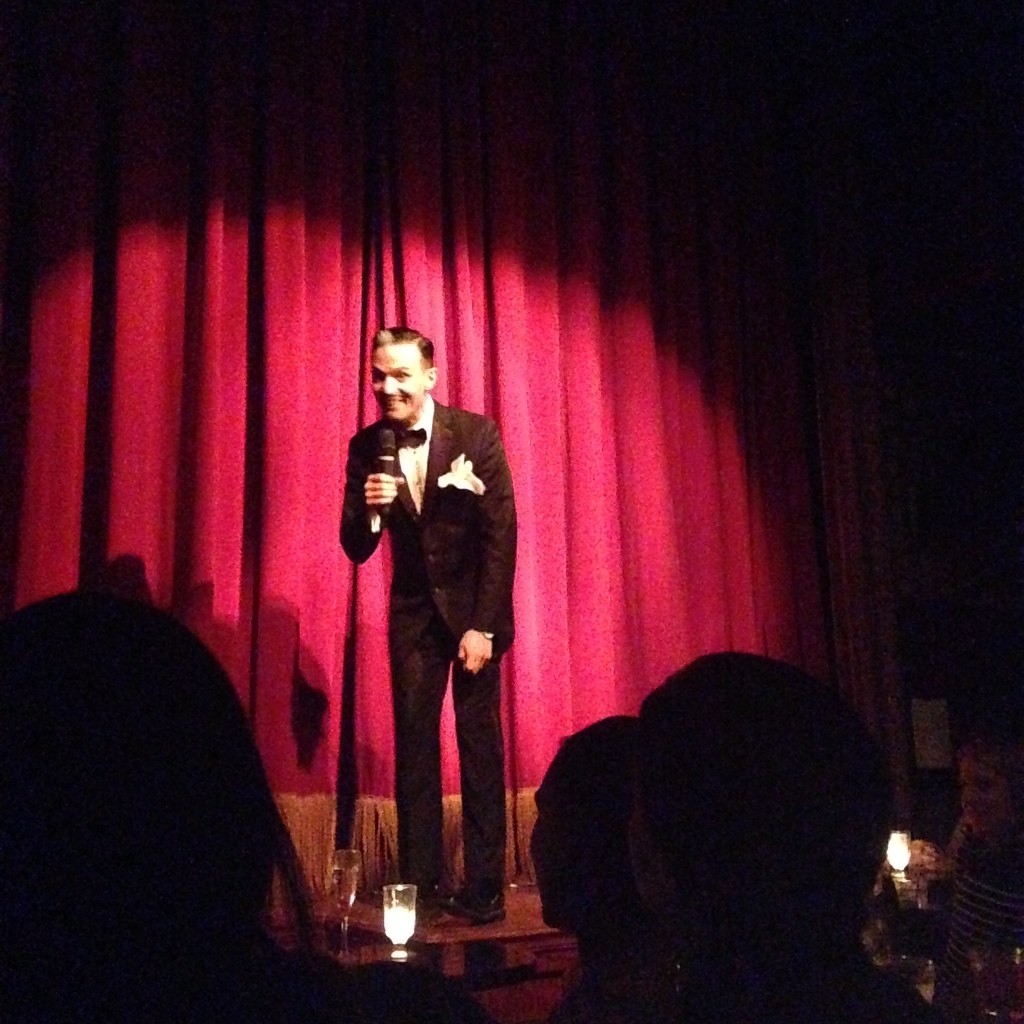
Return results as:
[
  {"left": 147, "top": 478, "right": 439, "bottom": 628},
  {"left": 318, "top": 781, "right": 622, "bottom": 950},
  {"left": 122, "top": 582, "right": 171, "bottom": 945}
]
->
[
  {"left": 341, "top": 327, "right": 516, "bottom": 926},
  {"left": 0, "top": 589, "right": 495, "bottom": 1024},
  {"left": 531, "top": 650, "right": 1023, "bottom": 1024}
]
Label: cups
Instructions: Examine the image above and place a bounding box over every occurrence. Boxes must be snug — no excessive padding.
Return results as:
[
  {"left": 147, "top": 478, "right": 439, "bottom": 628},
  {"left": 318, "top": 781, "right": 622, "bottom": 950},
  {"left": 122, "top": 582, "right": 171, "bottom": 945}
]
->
[
  {"left": 890, "top": 936, "right": 934, "bottom": 984},
  {"left": 967, "top": 943, "right": 1024, "bottom": 1024}
]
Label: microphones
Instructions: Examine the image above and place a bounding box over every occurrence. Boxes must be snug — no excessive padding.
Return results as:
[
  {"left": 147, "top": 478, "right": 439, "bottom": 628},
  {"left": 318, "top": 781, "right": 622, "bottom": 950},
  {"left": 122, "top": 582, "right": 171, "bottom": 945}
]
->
[{"left": 378, "top": 429, "right": 396, "bottom": 514}]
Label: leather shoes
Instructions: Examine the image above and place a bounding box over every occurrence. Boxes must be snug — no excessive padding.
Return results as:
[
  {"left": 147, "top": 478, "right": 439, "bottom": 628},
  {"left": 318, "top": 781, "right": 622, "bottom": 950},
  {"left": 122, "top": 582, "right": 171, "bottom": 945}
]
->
[{"left": 416, "top": 884, "right": 507, "bottom": 924}]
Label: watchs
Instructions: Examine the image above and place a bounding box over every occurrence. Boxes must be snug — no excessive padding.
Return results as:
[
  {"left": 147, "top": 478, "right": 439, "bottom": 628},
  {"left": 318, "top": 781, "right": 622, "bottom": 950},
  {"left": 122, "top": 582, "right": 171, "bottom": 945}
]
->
[{"left": 479, "top": 630, "right": 495, "bottom": 639}]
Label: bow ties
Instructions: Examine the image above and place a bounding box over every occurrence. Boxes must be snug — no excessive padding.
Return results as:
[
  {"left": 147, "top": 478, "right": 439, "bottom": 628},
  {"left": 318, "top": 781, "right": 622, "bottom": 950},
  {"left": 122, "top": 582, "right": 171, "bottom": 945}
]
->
[{"left": 391, "top": 427, "right": 427, "bottom": 450}]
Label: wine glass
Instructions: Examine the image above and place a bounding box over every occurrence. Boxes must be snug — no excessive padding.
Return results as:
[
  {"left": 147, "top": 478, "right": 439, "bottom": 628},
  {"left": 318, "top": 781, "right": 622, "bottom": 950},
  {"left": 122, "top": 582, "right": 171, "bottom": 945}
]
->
[
  {"left": 324, "top": 850, "right": 361, "bottom": 959},
  {"left": 384, "top": 883, "right": 417, "bottom": 961}
]
